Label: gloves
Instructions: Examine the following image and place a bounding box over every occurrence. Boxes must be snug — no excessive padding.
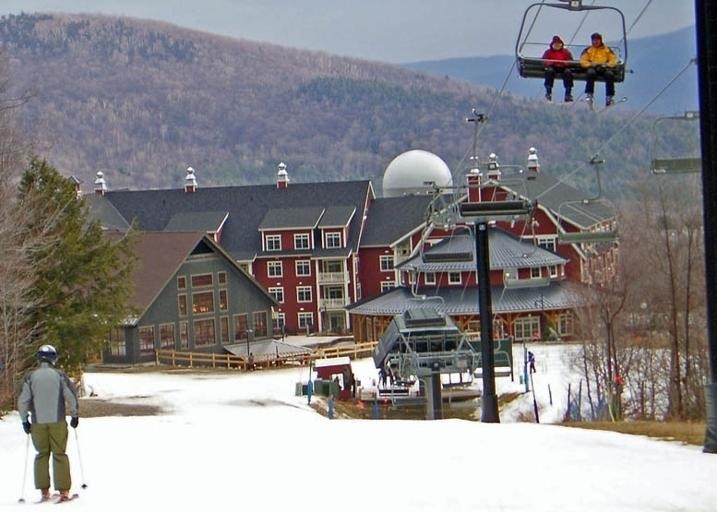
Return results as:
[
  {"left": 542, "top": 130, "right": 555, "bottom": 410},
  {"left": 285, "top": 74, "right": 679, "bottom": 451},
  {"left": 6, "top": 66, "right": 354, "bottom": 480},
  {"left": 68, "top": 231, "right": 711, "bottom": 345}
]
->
[
  {"left": 70, "top": 417, "right": 78, "bottom": 428},
  {"left": 23, "top": 421, "right": 31, "bottom": 434}
]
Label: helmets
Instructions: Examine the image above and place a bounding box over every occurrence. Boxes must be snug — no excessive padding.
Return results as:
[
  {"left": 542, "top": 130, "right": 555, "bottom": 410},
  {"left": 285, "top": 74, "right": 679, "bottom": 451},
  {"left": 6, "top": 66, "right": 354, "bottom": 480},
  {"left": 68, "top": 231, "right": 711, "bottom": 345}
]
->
[{"left": 37, "top": 345, "right": 57, "bottom": 366}]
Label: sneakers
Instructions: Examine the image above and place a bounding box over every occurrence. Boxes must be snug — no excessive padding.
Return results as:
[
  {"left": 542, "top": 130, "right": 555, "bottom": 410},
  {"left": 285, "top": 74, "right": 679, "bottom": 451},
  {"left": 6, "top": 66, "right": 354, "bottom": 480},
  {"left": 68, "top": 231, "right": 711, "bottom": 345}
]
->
[
  {"left": 545, "top": 94, "right": 551, "bottom": 101},
  {"left": 565, "top": 96, "right": 573, "bottom": 102},
  {"left": 585, "top": 93, "right": 594, "bottom": 103},
  {"left": 42, "top": 489, "right": 68, "bottom": 501},
  {"left": 606, "top": 96, "right": 614, "bottom": 106}
]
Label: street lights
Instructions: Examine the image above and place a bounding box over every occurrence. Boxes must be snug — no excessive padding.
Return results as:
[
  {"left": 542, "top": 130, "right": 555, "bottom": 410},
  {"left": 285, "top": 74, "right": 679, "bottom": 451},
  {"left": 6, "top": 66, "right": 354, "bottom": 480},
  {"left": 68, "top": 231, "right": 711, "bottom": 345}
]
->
[
  {"left": 246, "top": 325, "right": 250, "bottom": 357},
  {"left": 459, "top": 201, "right": 530, "bottom": 420}
]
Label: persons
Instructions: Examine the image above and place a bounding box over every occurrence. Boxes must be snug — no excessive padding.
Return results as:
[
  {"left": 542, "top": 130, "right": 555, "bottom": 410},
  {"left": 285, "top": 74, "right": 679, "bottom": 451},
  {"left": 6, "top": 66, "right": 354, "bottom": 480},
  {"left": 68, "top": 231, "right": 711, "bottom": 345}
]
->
[
  {"left": 578, "top": 33, "right": 617, "bottom": 107},
  {"left": 247, "top": 353, "right": 255, "bottom": 371},
  {"left": 541, "top": 35, "right": 574, "bottom": 103},
  {"left": 17, "top": 343, "right": 79, "bottom": 500},
  {"left": 526, "top": 351, "right": 535, "bottom": 374}
]
